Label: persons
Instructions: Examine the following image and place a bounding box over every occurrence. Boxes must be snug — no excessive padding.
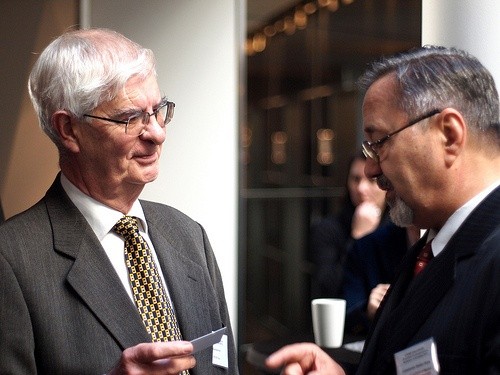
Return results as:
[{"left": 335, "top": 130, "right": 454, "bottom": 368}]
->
[
  {"left": 303, "top": 151, "right": 422, "bottom": 341},
  {"left": 265, "top": 43, "right": 500, "bottom": 375},
  {"left": 0, "top": 27, "right": 245, "bottom": 375}
]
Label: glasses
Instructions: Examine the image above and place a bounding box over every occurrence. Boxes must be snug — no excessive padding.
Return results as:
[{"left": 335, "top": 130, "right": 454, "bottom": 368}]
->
[
  {"left": 84, "top": 101, "right": 175, "bottom": 136},
  {"left": 362, "top": 109, "right": 441, "bottom": 159}
]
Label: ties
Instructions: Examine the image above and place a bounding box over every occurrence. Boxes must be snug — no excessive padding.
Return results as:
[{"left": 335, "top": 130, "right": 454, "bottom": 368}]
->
[
  {"left": 414, "top": 239, "right": 432, "bottom": 278},
  {"left": 112, "top": 215, "right": 189, "bottom": 375}
]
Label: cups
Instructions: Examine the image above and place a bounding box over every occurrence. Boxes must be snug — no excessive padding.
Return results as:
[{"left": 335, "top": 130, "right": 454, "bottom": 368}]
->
[{"left": 312, "top": 298, "right": 346, "bottom": 349}]
